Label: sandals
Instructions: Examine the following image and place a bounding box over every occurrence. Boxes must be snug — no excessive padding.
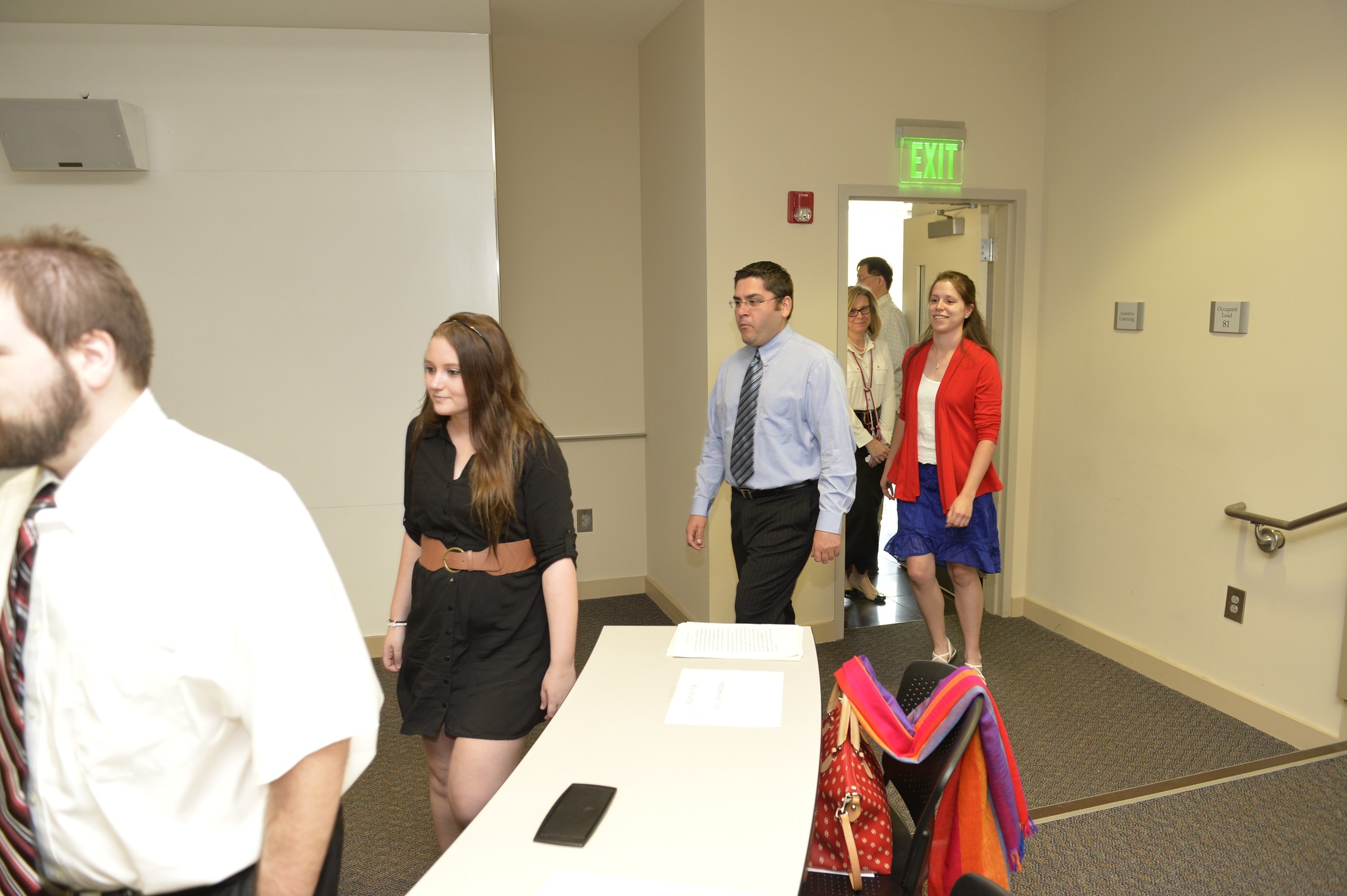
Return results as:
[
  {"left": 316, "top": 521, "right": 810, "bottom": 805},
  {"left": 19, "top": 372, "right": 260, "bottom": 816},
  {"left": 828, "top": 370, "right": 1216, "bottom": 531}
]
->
[
  {"left": 963, "top": 663, "right": 987, "bottom": 686},
  {"left": 931, "top": 637, "right": 957, "bottom": 664}
]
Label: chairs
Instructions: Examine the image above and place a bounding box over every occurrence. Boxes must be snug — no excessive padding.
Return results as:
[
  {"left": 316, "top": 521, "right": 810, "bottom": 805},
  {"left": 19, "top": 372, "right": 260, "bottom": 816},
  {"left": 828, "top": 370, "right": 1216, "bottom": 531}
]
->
[{"left": 808, "top": 659, "right": 984, "bottom": 896}]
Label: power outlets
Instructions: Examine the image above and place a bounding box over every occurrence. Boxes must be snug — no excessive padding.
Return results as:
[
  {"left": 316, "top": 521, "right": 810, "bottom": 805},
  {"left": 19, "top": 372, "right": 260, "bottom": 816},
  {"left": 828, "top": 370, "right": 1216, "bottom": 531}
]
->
[
  {"left": 1224, "top": 585, "right": 1247, "bottom": 625},
  {"left": 577, "top": 508, "right": 592, "bottom": 532}
]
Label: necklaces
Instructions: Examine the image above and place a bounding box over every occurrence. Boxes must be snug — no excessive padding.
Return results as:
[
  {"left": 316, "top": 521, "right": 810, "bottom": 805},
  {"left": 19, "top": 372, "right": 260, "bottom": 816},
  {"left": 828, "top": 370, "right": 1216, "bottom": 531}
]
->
[
  {"left": 932, "top": 342, "right": 955, "bottom": 368},
  {"left": 848, "top": 333, "right": 868, "bottom": 355}
]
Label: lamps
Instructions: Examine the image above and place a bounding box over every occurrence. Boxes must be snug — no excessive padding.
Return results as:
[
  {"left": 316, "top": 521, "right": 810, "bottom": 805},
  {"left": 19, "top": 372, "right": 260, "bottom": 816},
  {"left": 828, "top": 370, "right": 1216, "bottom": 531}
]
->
[{"left": 787, "top": 190, "right": 814, "bottom": 224}]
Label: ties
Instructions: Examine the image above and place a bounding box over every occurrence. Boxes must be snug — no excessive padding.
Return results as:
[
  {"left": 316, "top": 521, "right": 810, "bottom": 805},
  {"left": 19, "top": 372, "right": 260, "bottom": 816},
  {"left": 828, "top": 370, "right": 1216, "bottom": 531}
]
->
[
  {"left": 730, "top": 348, "right": 764, "bottom": 486},
  {"left": 0, "top": 482, "right": 58, "bottom": 896}
]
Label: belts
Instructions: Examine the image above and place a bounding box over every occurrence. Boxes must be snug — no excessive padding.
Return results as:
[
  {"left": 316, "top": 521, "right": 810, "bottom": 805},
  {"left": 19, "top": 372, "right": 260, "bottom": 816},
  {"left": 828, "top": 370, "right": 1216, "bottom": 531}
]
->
[
  {"left": 854, "top": 407, "right": 881, "bottom": 426},
  {"left": 417, "top": 535, "right": 536, "bottom": 577}
]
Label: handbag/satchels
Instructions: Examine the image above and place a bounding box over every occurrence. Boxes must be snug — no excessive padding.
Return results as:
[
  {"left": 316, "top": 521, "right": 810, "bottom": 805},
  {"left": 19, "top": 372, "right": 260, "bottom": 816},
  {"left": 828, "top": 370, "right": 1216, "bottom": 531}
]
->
[{"left": 803, "top": 681, "right": 893, "bottom": 891}]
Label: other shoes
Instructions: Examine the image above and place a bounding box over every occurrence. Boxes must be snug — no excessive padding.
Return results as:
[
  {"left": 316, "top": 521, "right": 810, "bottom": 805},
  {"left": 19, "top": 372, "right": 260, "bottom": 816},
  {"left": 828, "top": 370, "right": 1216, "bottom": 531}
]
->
[
  {"left": 848, "top": 573, "right": 886, "bottom": 601},
  {"left": 866, "top": 563, "right": 879, "bottom": 576},
  {"left": 844, "top": 579, "right": 864, "bottom": 600}
]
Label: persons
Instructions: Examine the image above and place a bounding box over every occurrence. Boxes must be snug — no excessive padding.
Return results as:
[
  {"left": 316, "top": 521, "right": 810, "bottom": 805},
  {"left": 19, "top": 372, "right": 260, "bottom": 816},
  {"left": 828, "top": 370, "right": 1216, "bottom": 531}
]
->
[
  {"left": 842, "top": 286, "right": 897, "bottom": 602},
  {"left": 854, "top": 258, "right": 910, "bottom": 572},
  {"left": 878, "top": 271, "right": 1005, "bottom": 689},
  {"left": 0, "top": 224, "right": 386, "bottom": 896},
  {"left": 685, "top": 260, "right": 857, "bottom": 628},
  {"left": 382, "top": 311, "right": 580, "bottom": 851}
]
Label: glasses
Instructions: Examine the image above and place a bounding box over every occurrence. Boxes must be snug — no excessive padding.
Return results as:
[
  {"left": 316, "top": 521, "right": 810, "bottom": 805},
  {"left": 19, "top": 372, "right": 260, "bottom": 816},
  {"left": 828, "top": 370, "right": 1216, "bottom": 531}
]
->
[
  {"left": 856, "top": 273, "right": 880, "bottom": 284},
  {"left": 848, "top": 306, "right": 871, "bottom": 317},
  {"left": 729, "top": 294, "right": 785, "bottom": 310}
]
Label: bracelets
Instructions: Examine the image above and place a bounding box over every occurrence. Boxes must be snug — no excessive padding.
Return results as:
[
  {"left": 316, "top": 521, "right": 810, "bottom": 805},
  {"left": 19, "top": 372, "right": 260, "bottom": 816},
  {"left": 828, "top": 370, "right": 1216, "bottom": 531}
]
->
[{"left": 388, "top": 618, "right": 408, "bottom": 630}]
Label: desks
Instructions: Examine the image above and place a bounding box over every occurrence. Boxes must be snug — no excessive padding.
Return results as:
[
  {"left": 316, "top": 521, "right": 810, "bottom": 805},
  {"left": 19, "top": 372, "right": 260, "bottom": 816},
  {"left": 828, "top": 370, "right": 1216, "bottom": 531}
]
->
[{"left": 402, "top": 625, "right": 822, "bottom": 896}]
